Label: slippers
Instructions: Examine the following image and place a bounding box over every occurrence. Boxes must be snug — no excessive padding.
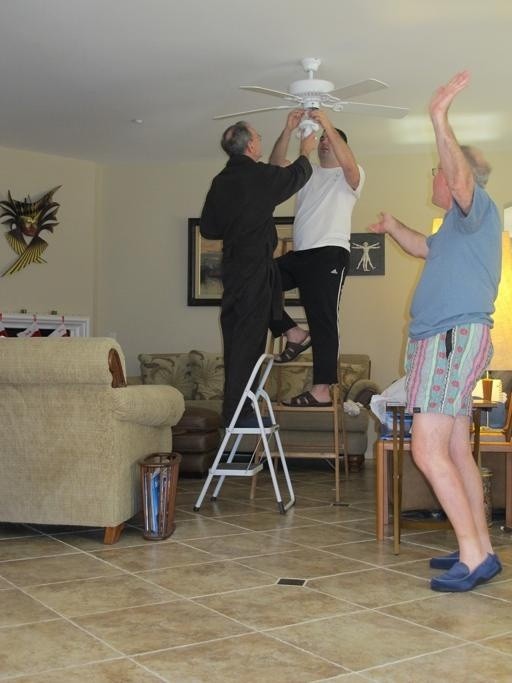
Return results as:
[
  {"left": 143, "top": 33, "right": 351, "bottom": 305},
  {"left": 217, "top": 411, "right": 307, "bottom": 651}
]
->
[
  {"left": 282, "top": 391, "right": 332, "bottom": 406},
  {"left": 273, "top": 331, "right": 312, "bottom": 362}
]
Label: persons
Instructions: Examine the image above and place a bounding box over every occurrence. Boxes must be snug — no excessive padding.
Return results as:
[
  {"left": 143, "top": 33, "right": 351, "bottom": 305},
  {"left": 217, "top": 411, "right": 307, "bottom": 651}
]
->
[
  {"left": 367, "top": 67, "right": 503, "bottom": 591},
  {"left": 198, "top": 121, "right": 319, "bottom": 427},
  {"left": 266, "top": 106, "right": 364, "bottom": 406}
]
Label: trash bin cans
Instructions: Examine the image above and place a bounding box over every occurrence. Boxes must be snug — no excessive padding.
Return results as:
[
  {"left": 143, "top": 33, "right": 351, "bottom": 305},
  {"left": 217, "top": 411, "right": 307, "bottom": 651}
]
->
[{"left": 138, "top": 452, "right": 182, "bottom": 541}]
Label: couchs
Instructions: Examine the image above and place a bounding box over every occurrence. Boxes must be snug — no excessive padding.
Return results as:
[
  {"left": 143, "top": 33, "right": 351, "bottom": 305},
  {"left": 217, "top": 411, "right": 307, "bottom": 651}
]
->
[
  {"left": 369, "top": 369, "right": 511, "bottom": 519},
  {"left": 137, "top": 350, "right": 384, "bottom": 476},
  {"left": 0, "top": 336, "right": 186, "bottom": 544}
]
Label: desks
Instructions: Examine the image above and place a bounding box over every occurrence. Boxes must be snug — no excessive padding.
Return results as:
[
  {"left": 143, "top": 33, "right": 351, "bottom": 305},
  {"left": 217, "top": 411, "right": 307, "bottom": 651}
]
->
[{"left": 385, "top": 398, "right": 499, "bottom": 554}]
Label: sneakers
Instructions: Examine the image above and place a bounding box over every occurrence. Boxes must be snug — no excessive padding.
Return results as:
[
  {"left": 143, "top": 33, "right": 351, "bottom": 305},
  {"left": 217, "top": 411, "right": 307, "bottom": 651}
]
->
[
  {"left": 429, "top": 550, "right": 501, "bottom": 591},
  {"left": 225, "top": 415, "right": 271, "bottom": 428}
]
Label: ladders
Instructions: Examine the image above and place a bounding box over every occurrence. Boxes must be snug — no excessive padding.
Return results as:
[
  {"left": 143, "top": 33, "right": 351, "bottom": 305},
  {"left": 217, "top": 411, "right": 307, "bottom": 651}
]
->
[
  {"left": 249, "top": 237, "right": 350, "bottom": 502},
  {"left": 193, "top": 353, "right": 297, "bottom": 515}
]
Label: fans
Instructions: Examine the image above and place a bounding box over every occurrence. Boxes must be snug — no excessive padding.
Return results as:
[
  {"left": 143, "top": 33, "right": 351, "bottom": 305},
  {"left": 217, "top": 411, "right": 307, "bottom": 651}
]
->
[{"left": 211, "top": 57, "right": 409, "bottom": 122}]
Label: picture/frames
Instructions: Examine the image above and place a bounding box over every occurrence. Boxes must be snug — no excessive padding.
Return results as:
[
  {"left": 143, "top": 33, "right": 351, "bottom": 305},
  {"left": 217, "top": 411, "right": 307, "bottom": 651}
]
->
[{"left": 188, "top": 217, "right": 303, "bottom": 307}]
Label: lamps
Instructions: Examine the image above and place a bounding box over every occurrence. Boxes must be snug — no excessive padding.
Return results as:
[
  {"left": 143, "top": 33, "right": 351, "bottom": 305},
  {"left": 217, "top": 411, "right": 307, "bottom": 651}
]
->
[{"left": 295, "top": 101, "right": 323, "bottom": 138}]
